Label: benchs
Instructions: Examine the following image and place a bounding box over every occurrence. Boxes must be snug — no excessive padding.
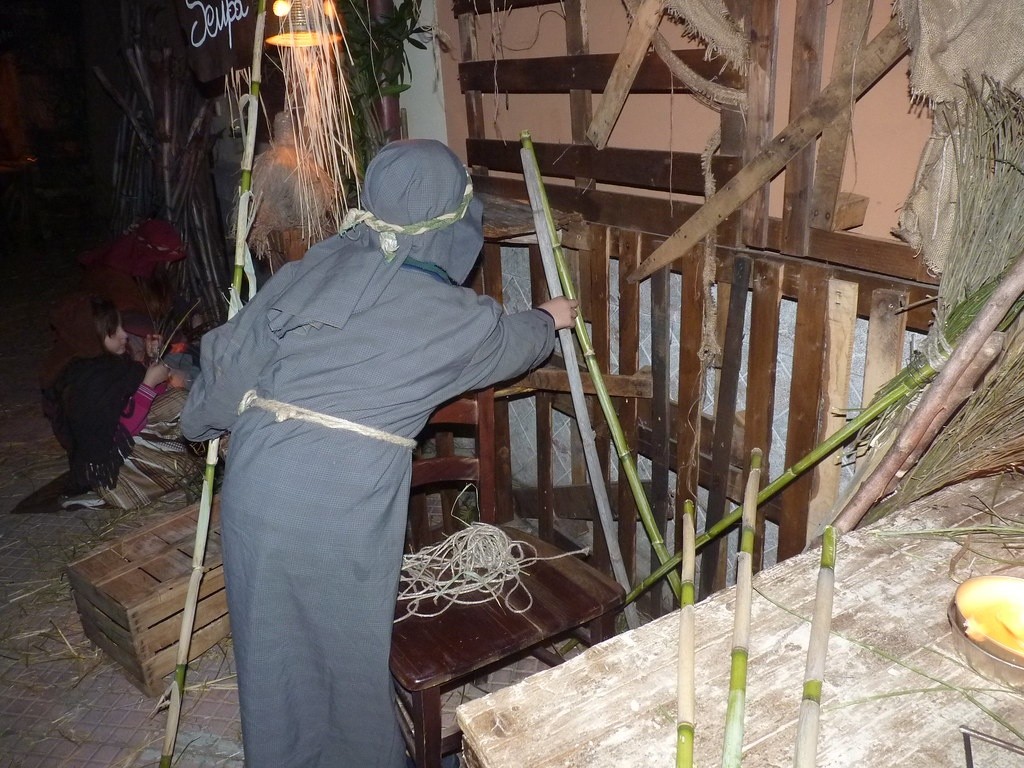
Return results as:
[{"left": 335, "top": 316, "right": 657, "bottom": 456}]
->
[{"left": 67, "top": 490, "right": 231, "bottom": 695}]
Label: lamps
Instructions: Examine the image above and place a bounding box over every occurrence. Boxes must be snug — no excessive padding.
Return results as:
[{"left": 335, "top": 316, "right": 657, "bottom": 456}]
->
[{"left": 260, "top": 0, "right": 343, "bottom": 47}]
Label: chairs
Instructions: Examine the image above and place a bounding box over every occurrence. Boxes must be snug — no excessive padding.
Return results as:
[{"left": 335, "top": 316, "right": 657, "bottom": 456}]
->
[{"left": 389, "top": 384, "right": 628, "bottom": 768}]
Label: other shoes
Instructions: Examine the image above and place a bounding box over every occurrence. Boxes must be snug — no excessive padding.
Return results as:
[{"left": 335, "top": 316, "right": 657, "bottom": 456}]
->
[{"left": 442, "top": 754, "right": 459, "bottom": 768}]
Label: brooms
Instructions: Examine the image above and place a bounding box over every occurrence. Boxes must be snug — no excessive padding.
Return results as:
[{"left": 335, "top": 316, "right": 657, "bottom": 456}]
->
[{"left": 557, "top": 66, "right": 1024, "bottom": 658}]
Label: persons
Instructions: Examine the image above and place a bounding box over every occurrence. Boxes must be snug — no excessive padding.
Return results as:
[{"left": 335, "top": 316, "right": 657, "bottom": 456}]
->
[
  {"left": 180, "top": 136, "right": 580, "bottom": 768},
  {"left": 48, "top": 292, "right": 206, "bottom": 512}
]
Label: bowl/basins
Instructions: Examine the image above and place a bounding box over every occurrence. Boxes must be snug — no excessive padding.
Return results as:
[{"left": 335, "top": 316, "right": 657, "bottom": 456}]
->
[{"left": 947, "top": 575, "right": 1024, "bottom": 692}]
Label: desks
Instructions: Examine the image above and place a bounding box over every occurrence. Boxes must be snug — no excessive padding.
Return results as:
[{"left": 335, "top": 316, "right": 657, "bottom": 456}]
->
[{"left": 456, "top": 466, "right": 1024, "bottom": 768}]
